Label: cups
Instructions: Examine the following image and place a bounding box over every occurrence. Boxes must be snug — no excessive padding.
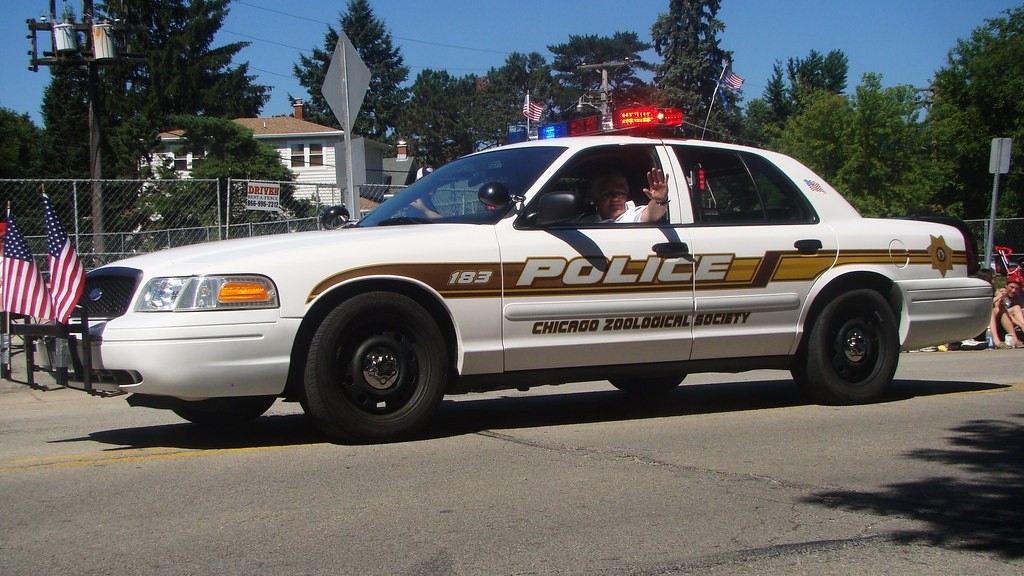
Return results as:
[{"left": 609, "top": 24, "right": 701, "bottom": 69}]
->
[{"left": 1005, "top": 334, "right": 1015, "bottom": 349}]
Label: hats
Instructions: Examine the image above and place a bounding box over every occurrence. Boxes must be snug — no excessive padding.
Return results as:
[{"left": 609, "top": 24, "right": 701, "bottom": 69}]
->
[{"left": 1005, "top": 275, "right": 1023, "bottom": 284}]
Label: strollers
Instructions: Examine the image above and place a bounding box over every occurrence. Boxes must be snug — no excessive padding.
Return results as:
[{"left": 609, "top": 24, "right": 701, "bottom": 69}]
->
[{"left": 995, "top": 246, "right": 1024, "bottom": 298}]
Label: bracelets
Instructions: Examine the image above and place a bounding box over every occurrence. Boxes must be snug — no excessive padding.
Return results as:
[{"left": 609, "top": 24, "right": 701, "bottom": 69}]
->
[{"left": 655, "top": 197, "right": 670, "bottom": 206}]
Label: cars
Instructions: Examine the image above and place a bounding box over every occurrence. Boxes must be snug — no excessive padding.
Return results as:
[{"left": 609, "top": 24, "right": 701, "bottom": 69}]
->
[{"left": 35, "top": 100, "right": 994, "bottom": 443}]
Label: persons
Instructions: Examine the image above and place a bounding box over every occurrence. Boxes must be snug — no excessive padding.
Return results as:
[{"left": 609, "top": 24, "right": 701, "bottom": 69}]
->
[
  {"left": 409, "top": 164, "right": 672, "bottom": 224},
  {"left": 908, "top": 266, "right": 1024, "bottom": 351}
]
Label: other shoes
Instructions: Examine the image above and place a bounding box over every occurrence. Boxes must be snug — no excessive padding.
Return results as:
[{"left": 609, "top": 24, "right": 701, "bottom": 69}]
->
[
  {"left": 994, "top": 342, "right": 1012, "bottom": 350},
  {"left": 919, "top": 346, "right": 938, "bottom": 353},
  {"left": 1015, "top": 340, "right": 1024, "bottom": 350},
  {"left": 961, "top": 340, "right": 988, "bottom": 350}
]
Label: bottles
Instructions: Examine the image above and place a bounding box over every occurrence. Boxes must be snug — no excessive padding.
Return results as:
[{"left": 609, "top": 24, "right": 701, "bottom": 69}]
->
[
  {"left": 993, "top": 289, "right": 1011, "bottom": 306},
  {"left": 985, "top": 326, "right": 994, "bottom": 351}
]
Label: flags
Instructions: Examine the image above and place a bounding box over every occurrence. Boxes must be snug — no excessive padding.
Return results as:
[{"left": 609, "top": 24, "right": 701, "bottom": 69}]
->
[
  {"left": 523, "top": 94, "right": 544, "bottom": 121},
  {"left": 43, "top": 192, "right": 86, "bottom": 325},
  {"left": 0, "top": 214, "right": 55, "bottom": 323},
  {"left": 719, "top": 65, "right": 744, "bottom": 91}
]
